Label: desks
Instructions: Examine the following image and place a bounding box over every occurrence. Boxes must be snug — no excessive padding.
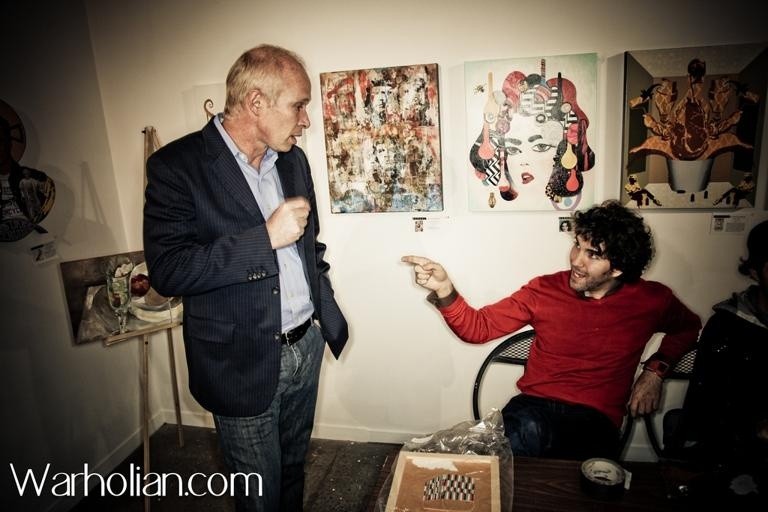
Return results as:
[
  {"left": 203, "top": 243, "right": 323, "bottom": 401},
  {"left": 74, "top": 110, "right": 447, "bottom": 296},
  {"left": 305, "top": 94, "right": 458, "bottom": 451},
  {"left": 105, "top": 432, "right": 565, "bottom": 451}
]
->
[{"left": 498, "top": 451, "right": 767, "bottom": 512}]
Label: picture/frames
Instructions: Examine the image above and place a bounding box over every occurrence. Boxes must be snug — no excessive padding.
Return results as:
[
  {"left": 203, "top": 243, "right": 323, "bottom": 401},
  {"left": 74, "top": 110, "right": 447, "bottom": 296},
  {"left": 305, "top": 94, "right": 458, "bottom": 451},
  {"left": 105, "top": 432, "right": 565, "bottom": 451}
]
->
[{"left": 386, "top": 447, "right": 501, "bottom": 511}]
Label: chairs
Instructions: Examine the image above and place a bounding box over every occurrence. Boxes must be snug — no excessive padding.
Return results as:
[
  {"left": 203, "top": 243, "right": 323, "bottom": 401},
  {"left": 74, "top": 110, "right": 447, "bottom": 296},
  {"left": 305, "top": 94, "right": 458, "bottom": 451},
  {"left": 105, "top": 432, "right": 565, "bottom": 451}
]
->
[
  {"left": 648, "top": 342, "right": 702, "bottom": 459},
  {"left": 476, "top": 328, "right": 634, "bottom": 458}
]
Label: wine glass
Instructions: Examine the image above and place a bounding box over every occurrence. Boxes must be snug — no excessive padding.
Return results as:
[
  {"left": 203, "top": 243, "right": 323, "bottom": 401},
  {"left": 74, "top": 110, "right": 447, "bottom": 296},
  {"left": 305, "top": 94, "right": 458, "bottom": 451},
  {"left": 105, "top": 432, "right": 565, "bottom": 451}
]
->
[{"left": 100, "top": 255, "right": 133, "bottom": 335}]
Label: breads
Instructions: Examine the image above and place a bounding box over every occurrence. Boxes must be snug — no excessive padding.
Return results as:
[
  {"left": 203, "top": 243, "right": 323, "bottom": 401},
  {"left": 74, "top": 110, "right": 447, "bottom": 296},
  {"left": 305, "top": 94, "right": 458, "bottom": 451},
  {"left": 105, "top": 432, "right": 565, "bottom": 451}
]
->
[{"left": 144, "top": 286, "right": 169, "bottom": 306}]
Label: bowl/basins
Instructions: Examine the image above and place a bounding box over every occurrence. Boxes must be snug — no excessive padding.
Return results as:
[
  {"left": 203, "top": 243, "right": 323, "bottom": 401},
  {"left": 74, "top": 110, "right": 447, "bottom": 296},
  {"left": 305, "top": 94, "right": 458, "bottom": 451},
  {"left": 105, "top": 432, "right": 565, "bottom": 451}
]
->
[{"left": 130, "top": 261, "right": 177, "bottom": 311}]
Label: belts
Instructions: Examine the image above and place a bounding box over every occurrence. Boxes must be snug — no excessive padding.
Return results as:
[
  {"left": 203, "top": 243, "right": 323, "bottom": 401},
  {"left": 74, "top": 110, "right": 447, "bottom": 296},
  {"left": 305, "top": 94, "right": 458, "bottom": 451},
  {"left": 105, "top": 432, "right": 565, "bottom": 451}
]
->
[
  {"left": 281, "top": 310, "right": 316, "bottom": 347},
  {"left": 520, "top": 394, "right": 588, "bottom": 415}
]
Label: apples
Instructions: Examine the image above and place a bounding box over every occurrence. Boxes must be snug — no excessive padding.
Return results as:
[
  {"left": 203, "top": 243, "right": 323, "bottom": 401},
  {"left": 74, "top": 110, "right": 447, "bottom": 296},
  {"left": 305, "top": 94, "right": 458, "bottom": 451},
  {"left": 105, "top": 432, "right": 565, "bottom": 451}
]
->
[{"left": 129, "top": 273, "right": 150, "bottom": 298}]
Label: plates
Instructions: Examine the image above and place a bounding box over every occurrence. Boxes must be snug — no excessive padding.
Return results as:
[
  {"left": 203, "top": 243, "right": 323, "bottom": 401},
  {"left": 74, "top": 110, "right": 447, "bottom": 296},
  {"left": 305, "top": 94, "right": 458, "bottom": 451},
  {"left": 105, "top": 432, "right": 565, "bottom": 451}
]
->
[{"left": 128, "top": 296, "right": 184, "bottom": 324}]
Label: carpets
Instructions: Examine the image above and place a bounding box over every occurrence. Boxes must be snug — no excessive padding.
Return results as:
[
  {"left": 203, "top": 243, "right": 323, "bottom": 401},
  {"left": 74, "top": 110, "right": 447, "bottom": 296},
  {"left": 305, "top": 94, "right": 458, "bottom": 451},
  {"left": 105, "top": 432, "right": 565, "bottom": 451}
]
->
[{"left": 75, "top": 422, "right": 392, "bottom": 512}]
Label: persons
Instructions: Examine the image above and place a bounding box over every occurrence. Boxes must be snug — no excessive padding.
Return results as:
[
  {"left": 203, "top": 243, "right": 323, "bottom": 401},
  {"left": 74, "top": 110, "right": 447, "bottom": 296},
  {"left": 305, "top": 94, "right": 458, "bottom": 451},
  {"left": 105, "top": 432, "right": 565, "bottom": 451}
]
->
[
  {"left": 401, "top": 201, "right": 702, "bottom": 462},
  {"left": 344, "top": 68, "right": 429, "bottom": 214},
  {"left": 145, "top": 44, "right": 349, "bottom": 509},
  {"left": 467, "top": 61, "right": 599, "bottom": 203},
  {"left": 683, "top": 224, "right": 768, "bottom": 460}
]
